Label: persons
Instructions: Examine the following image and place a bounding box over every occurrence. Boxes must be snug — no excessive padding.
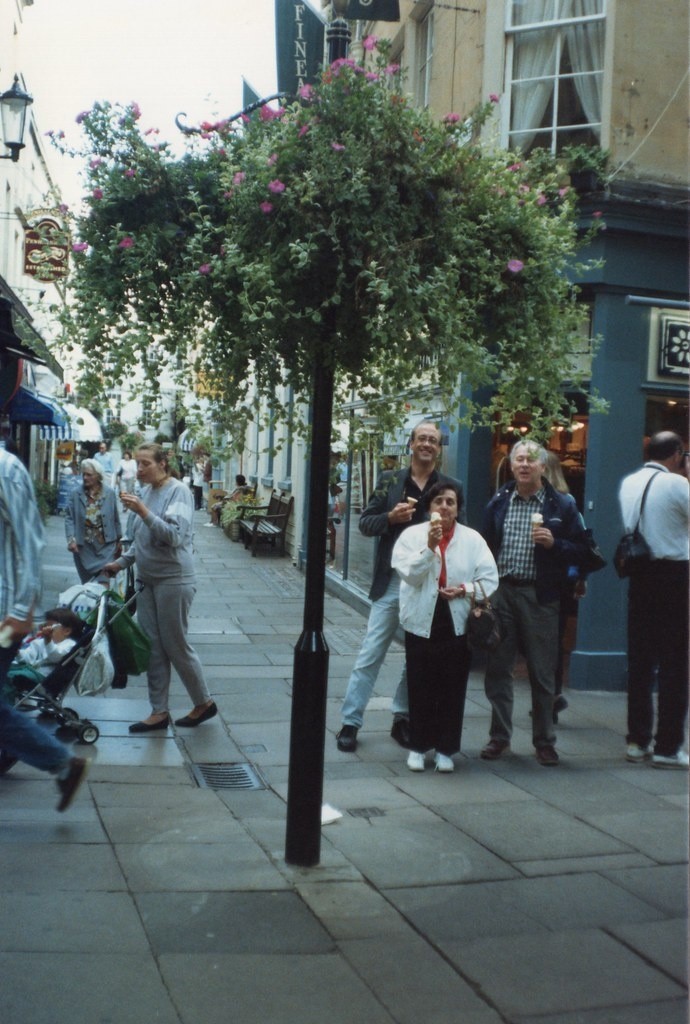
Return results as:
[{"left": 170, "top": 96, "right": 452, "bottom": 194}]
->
[
  {"left": 0, "top": 609, "right": 82, "bottom": 695},
  {"left": 0, "top": 447, "right": 91, "bottom": 814},
  {"left": 477, "top": 440, "right": 583, "bottom": 765},
  {"left": 93, "top": 443, "right": 115, "bottom": 487},
  {"left": 163, "top": 449, "right": 184, "bottom": 481},
  {"left": 206, "top": 475, "right": 245, "bottom": 527},
  {"left": 193, "top": 461, "right": 205, "bottom": 511},
  {"left": 529, "top": 451, "right": 585, "bottom": 724},
  {"left": 389, "top": 482, "right": 498, "bottom": 772},
  {"left": 339, "top": 420, "right": 462, "bottom": 751},
  {"left": 65, "top": 459, "right": 122, "bottom": 591},
  {"left": 200, "top": 452, "right": 212, "bottom": 510},
  {"left": 115, "top": 451, "right": 137, "bottom": 513},
  {"left": 103, "top": 443, "right": 218, "bottom": 733},
  {"left": 618, "top": 431, "right": 690, "bottom": 770}
]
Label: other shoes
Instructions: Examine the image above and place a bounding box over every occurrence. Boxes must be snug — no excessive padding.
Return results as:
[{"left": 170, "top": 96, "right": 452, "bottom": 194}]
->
[
  {"left": 129, "top": 715, "right": 171, "bottom": 733},
  {"left": 652, "top": 749, "right": 689, "bottom": 770},
  {"left": 175, "top": 703, "right": 218, "bottom": 728},
  {"left": 58, "top": 755, "right": 91, "bottom": 810},
  {"left": 626, "top": 743, "right": 655, "bottom": 761},
  {"left": 536, "top": 744, "right": 559, "bottom": 763},
  {"left": 481, "top": 739, "right": 510, "bottom": 758},
  {"left": 529, "top": 695, "right": 569, "bottom": 723},
  {"left": 203, "top": 522, "right": 217, "bottom": 528}
]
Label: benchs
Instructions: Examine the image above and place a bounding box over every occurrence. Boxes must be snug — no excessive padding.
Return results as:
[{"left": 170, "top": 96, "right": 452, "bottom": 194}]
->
[
  {"left": 236, "top": 489, "right": 294, "bottom": 558},
  {"left": 325, "top": 516, "right": 341, "bottom": 560}
]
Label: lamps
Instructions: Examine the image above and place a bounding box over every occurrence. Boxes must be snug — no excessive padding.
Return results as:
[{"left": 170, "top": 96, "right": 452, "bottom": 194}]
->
[{"left": 0, "top": 74, "right": 33, "bottom": 162}]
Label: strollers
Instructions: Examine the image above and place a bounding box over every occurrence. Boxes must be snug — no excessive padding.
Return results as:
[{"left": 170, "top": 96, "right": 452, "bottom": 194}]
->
[{"left": 7, "top": 567, "right": 144, "bottom": 745}]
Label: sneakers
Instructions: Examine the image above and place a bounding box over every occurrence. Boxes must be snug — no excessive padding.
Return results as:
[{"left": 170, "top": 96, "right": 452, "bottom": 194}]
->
[
  {"left": 406, "top": 751, "right": 425, "bottom": 772},
  {"left": 432, "top": 751, "right": 455, "bottom": 772},
  {"left": 335, "top": 725, "right": 360, "bottom": 751},
  {"left": 389, "top": 714, "right": 409, "bottom": 746}
]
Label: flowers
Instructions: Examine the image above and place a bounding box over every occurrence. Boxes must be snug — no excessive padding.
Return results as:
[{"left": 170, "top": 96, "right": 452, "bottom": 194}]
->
[
  {"left": 14, "top": 36, "right": 613, "bottom": 515},
  {"left": 220, "top": 487, "right": 267, "bottom": 528}
]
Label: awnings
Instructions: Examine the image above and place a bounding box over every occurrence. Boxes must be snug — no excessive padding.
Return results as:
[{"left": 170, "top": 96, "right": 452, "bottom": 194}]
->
[
  {"left": 39, "top": 403, "right": 103, "bottom": 442},
  {"left": 0, "top": 297, "right": 64, "bottom": 385},
  {"left": 8, "top": 385, "right": 66, "bottom": 426}
]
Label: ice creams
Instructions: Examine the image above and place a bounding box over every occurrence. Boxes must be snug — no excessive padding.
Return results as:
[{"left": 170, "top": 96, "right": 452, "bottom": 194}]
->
[
  {"left": 430, "top": 512, "right": 442, "bottom": 526},
  {"left": 407, "top": 497, "right": 418, "bottom": 509},
  {"left": 531, "top": 513, "right": 543, "bottom": 544}
]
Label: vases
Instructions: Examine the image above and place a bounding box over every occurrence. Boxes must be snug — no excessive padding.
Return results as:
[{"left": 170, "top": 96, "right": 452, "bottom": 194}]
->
[{"left": 221, "top": 517, "right": 241, "bottom": 542}]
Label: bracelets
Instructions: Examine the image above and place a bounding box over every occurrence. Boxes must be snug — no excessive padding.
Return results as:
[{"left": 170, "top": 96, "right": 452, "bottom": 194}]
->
[{"left": 458, "top": 587, "right": 465, "bottom": 598}]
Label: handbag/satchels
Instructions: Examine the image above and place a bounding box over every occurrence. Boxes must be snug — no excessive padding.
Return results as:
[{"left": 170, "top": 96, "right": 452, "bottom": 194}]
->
[
  {"left": 57, "top": 566, "right": 151, "bottom": 698},
  {"left": 575, "top": 526, "right": 606, "bottom": 578},
  {"left": 614, "top": 533, "right": 652, "bottom": 579},
  {"left": 464, "top": 580, "right": 505, "bottom": 656}
]
